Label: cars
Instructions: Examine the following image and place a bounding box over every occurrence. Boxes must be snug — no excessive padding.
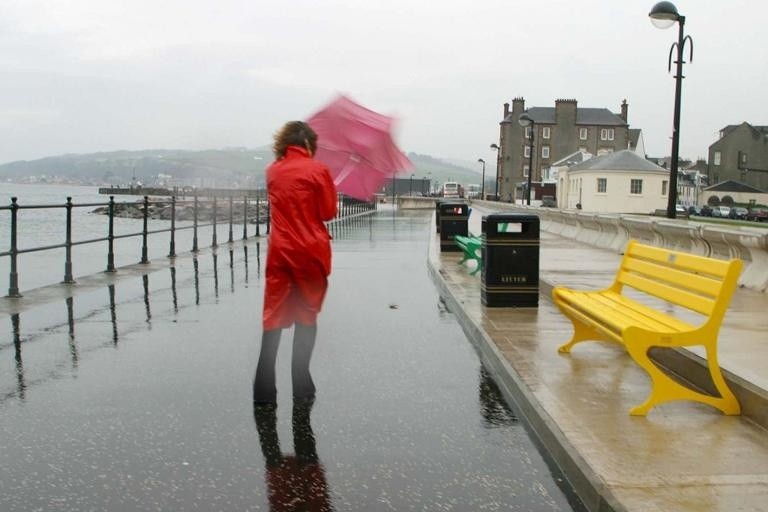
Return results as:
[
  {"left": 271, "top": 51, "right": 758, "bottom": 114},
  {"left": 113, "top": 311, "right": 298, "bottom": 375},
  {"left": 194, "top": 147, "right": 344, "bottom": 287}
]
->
[{"left": 674, "top": 204, "right": 768, "bottom": 222}]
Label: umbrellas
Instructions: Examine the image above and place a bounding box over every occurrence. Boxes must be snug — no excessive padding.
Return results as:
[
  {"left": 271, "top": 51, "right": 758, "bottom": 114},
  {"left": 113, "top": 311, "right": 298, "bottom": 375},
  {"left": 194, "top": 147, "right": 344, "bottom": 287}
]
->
[{"left": 307, "top": 95, "right": 415, "bottom": 202}]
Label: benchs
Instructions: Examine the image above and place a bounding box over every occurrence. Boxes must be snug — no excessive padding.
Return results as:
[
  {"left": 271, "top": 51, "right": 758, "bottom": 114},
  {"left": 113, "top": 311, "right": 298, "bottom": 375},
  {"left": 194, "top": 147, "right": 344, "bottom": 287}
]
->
[
  {"left": 454, "top": 209, "right": 511, "bottom": 277},
  {"left": 549, "top": 239, "right": 745, "bottom": 422}
]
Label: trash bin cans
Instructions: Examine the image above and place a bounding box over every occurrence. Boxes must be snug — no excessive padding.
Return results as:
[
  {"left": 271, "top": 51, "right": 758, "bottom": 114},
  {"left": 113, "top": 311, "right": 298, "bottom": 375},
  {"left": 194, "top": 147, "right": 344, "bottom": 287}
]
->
[
  {"left": 440, "top": 203, "right": 468, "bottom": 252},
  {"left": 481, "top": 214, "right": 540, "bottom": 307},
  {"left": 436, "top": 202, "right": 447, "bottom": 233},
  {"left": 543, "top": 196, "right": 553, "bottom": 207}
]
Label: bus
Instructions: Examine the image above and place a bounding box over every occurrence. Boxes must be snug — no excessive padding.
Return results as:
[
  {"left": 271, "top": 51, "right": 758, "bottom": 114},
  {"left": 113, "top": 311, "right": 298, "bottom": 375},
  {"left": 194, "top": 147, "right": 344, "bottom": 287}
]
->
[{"left": 441, "top": 182, "right": 459, "bottom": 198}]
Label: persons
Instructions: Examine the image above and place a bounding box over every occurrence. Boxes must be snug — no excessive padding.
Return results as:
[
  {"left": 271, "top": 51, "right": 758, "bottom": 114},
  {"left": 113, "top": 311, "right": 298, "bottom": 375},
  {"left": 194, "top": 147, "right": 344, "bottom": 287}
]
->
[{"left": 254, "top": 122, "right": 337, "bottom": 399}]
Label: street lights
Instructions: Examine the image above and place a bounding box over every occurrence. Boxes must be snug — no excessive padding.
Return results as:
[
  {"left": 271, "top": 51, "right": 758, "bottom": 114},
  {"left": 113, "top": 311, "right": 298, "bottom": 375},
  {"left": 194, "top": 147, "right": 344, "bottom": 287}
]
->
[
  {"left": 648, "top": 0, "right": 693, "bottom": 220},
  {"left": 489, "top": 142, "right": 501, "bottom": 202},
  {"left": 477, "top": 159, "right": 485, "bottom": 201},
  {"left": 391, "top": 169, "right": 439, "bottom": 207},
  {"left": 516, "top": 111, "right": 535, "bottom": 207}
]
